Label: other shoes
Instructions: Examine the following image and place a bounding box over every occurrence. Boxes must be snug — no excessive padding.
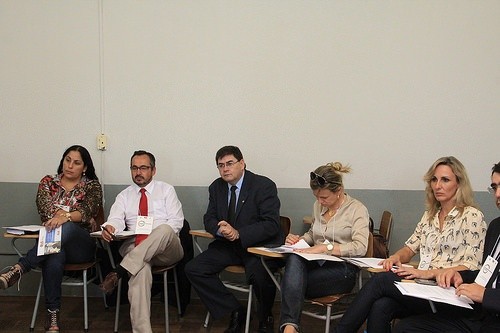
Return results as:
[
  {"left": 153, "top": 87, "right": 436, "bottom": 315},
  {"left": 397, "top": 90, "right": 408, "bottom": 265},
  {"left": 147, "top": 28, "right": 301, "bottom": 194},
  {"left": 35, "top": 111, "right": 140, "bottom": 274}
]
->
[{"left": 100, "top": 272, "right": 118, "bottom": 296}]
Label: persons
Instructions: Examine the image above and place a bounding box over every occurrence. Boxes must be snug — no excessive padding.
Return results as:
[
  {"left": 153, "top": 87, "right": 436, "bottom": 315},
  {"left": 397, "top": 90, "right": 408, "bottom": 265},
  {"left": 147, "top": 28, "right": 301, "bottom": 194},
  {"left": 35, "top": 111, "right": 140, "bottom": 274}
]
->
[
  {"left": 333, "top": 156, "right": 488, "bottom": 333},
  {"left": 101, "top": 149, "right": 184, "bottom": 333},
  {"left": 0, "top": 145, "right": 104, "bottom": 333},
  {"left": 392, "top": 161, "right": 500, "bottom": 333},
  {"left": 280, "top": 161, "right": 370, "bottom": 333},
  {"left": 185, "top": 146, "right": 287, "bottom": 333}
]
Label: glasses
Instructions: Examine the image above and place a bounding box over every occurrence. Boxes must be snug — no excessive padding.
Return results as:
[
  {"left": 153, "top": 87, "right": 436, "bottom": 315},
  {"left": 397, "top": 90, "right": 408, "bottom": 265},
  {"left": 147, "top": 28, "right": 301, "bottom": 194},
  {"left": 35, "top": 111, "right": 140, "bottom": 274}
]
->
[
  {"left": 130, "top": 166, "right": 153, "bottom": 171},
  {"left": 487, "top": 185, "right": 500, "bottom": 194},
  {"left": 310, "top": 172, "right": 341, "bottom": 187},
  {"left": 217, "top": 159, "right": 241, "bottom": 169}
]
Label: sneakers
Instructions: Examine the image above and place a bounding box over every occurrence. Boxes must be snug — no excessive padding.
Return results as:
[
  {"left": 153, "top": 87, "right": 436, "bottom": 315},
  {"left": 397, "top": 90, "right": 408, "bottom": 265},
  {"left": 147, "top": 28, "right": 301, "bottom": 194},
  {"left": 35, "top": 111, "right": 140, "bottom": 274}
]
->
[
  {"left": 44, "top": 304, "right": 60, "bottom": 333},
  {"left": 0, "top": 264, "right": 23, "bottom": 292}
]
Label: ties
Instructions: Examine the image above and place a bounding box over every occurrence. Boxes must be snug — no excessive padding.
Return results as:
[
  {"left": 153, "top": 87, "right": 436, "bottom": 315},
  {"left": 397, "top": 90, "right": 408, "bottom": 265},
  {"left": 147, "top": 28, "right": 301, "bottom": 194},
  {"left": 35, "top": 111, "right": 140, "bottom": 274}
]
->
[
  {"left": 228, "top": 186, "right": 238, "bottom": 227},
  {"left": 135, "top": 188, "right": 149, "bottom": 247}
]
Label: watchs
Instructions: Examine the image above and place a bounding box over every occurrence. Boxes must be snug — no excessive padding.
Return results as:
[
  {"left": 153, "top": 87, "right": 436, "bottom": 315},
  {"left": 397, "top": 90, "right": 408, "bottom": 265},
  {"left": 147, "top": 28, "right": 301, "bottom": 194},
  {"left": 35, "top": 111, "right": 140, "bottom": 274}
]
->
[
  {"left": 62, "top": 213, "right": 72, "bottom": 221},
  {"left": 327, "top": 243, "right": 333, "bottom": 254}
]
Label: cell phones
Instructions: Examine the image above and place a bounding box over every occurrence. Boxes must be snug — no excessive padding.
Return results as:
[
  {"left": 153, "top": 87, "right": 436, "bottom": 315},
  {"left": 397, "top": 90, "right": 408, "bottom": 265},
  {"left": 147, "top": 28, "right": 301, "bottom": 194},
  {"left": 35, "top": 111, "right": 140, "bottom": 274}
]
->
[{"left": 414, "top": 278, "right": 438, "bottom": 285}]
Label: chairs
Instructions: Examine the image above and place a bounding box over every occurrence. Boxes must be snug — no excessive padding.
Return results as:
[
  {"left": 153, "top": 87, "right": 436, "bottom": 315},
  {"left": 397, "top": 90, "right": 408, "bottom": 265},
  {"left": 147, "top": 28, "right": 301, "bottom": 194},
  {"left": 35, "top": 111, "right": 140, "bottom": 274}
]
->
[{"left": 9, "top": 211, "right": 417, "bottom": 333}]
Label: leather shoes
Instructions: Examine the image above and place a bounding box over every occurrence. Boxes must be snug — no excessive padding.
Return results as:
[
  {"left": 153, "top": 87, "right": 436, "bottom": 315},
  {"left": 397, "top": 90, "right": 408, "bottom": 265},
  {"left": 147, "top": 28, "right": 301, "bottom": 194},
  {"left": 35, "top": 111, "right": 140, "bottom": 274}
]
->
[
  {"left": 258, "top": 315, "right": 274, "bottom": 333},
  {"left": 224, "top": 308, "right": 246, "bottom": 333}
]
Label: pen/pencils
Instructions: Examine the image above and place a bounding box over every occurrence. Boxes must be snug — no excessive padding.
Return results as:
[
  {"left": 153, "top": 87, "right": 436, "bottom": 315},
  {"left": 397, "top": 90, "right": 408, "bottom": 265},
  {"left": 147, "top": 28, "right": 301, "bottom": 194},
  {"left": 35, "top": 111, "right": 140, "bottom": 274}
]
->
[
  {"left": 392, "top": 266, "right": 399, "bottom": 269},
  {"left": 100, "top": 225, "right": 116, "bottom": 239}
]
